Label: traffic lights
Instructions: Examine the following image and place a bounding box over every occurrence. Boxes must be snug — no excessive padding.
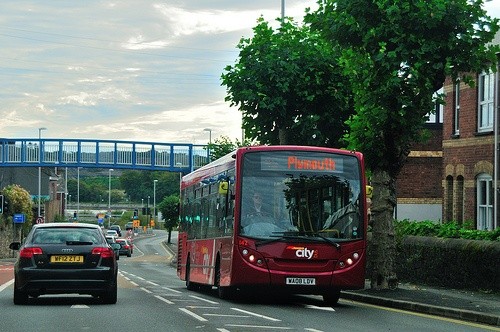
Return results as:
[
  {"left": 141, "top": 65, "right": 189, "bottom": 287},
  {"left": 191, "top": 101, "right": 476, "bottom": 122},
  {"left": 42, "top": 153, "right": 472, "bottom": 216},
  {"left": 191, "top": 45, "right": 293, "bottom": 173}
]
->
[
  {"left": 142, "top": 208, "right": 146, "bottom": 215},
  {"left": 0, "top": 195, "right": 8, "bottom": 215},
  {"left": 41, "top": 206, "right": 45, "bottom": 217}
]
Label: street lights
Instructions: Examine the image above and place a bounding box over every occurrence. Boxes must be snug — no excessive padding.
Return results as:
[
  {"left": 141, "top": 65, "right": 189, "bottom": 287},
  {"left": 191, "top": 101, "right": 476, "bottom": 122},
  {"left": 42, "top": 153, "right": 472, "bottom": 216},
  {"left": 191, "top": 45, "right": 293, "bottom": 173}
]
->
[
  {"left": 76, "top": 166, "right": 84, "bottom": 219},
  {"left": 203, "top": 128, "right": 212, "bottom": 161},
  {"left": 108, "top": 169, "right": 114, "bottom": 227},
  {"left": 154, "top": 179, "right": 159, "bottom": 216},
  {"left": 64, "top": 149, "right": 73, "bottom": 217},
  {"left": 176, "top": 162, "right": 182, "bottom": 196},
  {"left": 37, "top": 127, "right": 48, "bottom": 216}
]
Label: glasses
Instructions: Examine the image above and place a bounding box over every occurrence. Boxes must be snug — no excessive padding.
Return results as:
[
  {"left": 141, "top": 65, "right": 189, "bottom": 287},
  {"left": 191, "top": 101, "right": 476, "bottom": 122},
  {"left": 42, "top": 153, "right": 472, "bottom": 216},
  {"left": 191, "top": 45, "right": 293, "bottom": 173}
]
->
[{"left": 254, "top": 196, "right": 262, "bottom": 199}]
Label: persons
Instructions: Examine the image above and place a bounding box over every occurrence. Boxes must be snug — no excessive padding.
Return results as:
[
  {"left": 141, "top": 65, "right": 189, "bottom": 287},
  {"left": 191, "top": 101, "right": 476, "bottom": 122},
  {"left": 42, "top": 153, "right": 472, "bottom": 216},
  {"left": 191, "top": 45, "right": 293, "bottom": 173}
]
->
[{"left": 243, "top": 191, "right": 272, "bottom": 225}]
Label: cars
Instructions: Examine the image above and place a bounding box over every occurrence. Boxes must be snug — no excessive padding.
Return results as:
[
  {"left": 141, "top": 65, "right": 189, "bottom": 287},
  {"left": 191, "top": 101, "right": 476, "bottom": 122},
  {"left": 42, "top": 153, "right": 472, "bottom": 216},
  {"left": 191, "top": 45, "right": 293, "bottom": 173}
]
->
[
  {"left": 8, "top": 222, "right": 118, "bottom": 305},
  {"left": 105, "top": 222, "right": 134, "bottom": 260}
]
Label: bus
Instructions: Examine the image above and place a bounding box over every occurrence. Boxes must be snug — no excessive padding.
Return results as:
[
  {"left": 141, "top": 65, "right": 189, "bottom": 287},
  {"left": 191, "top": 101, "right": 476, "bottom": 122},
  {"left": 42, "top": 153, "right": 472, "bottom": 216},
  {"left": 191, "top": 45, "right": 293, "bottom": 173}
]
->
[{"left": 177, "top": 144, "right": 367, "bottom": 304}]
